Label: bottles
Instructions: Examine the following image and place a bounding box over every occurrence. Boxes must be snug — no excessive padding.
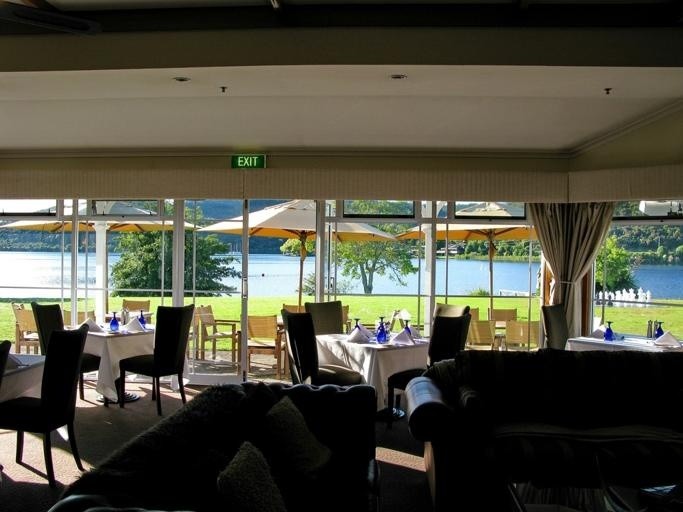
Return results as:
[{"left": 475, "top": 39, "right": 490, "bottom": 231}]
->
[{"left": 647, "top": 320, "right": 658, "bottom": 338}]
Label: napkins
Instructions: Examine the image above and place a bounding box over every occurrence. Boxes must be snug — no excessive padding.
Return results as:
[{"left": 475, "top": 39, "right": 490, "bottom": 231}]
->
[
  {"left": 345, "top": 328, "right": 369, "bottom": 343},
  {"left": 394, "top": 329, "right": 414, "bottom": 346},
  {"left": 121, "top": 319, "right": 146, "bottom": 331},
  {"left": 408, "top": 325, "right": 422, "bottom": 337},
  {"left": 351, "top": 323, "right": 373, "bottom": 336},
  {"left": 8, "top": 354, "right": 25, "bottom": 367},
  {"left": 75, "top": 319, "right": 104, "bottom": 332}
]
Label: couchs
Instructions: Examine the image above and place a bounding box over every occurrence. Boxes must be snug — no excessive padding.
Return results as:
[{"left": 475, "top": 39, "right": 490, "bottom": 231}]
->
[
  {"left": 47, "top": 382, "right": 379, "bottom": 512},
  {"left": 399, "top": 349, "right": 683, "bottom": 512}
]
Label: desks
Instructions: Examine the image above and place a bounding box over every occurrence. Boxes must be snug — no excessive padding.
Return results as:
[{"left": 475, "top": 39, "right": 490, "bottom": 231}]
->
[{"left": 565, "top": 331, "right": 683, "bottom": 353}]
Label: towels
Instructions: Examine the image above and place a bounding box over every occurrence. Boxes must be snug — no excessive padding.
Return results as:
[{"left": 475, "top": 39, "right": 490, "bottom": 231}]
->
[
  {"left": 587, "top": 323, "right": 608, "bottom": 338},
  {"left": 652, "top": 331, "right": 683, "bottom": 347}
]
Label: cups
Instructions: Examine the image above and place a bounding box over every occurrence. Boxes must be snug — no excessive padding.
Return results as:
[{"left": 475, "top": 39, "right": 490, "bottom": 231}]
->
[{"left": 121, "top": 308, "right": 130, "bottom": 325}]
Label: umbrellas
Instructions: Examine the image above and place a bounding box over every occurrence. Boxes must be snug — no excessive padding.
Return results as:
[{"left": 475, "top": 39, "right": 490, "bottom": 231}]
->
[
  {"left": 396, "top": 202, "right": 542, "bottom": 320},
  {"left": 195, "top": 199, "right": 399, "bottom": 313},
  {"left": 0, "top": 200, "right": 201, "bottom": 323}
]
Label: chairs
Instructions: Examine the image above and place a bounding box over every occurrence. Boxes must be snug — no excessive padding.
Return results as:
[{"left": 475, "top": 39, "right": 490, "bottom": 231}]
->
[
  {"left": 120, "top": 301, "right": 151, "bottom": 325},
  {"left": 115, "top": 305, "right": 192, "bottom": 414},
  {"left": 1, "top": 326, "right": 88, "bottom": 490},
  {"left": 12, "top": 304, "right": 39, "bottom": 355},
  {"left": 35, "top": 302, "right": 109, "bottom": 408},
  {"left": 195, "top": 304, "right": 240, "bottom": 364},
  {"left": 305, "top": 299, "right": 345, "bottom": 335},
  {"left": 186, "top": 309, "right": 201, "bottom": 362},
  {"left": 2, "top": 339, "right": 11, "bottom": 382},
  {"left": 62, "top": 310, "right": 95, "bottom": 328},
  {"left": 280, "top": 310, "right": 362, "bottom": 386},
  {"left": 389, "top": 311, "right": 472, "bottom": 412},
  {"left": 429, "top": 302, "right": 467, "bottom": 350},
  {"left": 284, "top": 304, "right": 353, "bottom": 325},
  {"left": 240, "top": 314, "right": 288, "bottom": 380},
  {"left": 467, "top": 307, "right": 571, "bottom": 347},
  {"left": 541, "top": 302, "right": 570, "bottom": 349}
]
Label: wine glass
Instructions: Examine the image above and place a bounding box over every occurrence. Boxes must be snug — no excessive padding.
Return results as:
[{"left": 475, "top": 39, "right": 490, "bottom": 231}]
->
[
  {"left": 110, "top": 311, "right": 119, "bottom": 331},
  {"left": 404, "top": 319, "right": 412, "bottom": 336},
  {"left": 655, "top": 322, "right": 665, "bottom": 338},
  {"left": 352, "top": 318, "right": 361, "bottom": 330},
  {"left": 139, "top": 309, "right": 146, "bottom": 330},
  {"left": 377, "top": 317, "right": 387, "bottom": 343},
  {"left": 604, "top": 321, "right": 616, "bottom": 341}
]
